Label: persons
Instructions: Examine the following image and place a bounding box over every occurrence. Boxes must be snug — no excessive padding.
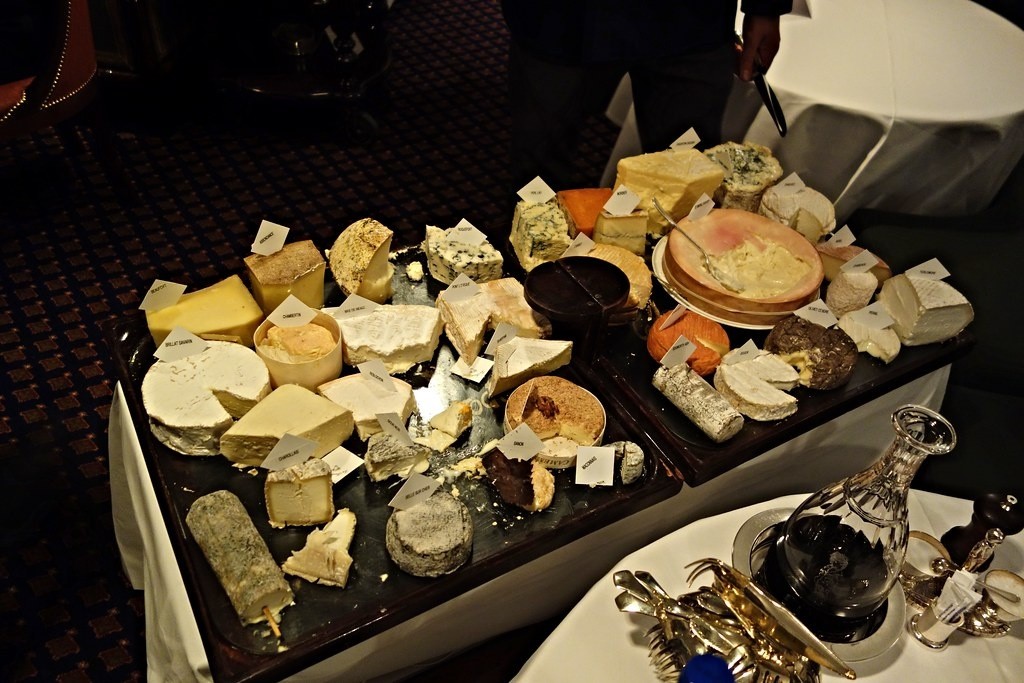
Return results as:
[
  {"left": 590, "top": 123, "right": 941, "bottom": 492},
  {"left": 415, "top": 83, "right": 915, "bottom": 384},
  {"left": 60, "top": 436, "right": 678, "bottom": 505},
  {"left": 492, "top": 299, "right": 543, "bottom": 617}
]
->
[{"left": 501, "top": 0, "right": 794, "bottom": 193}]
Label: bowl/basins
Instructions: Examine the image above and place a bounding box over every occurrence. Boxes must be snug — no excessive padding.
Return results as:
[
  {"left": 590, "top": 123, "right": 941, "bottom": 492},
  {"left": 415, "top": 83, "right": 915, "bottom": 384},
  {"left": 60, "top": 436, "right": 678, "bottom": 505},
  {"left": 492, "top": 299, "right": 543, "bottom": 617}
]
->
[
  {"left": 899, "top": 529, "right": 952, "bottom": 578},
  {"left": 504, "top": 376, "right": 606, "bottom": 469},
  {"left": 983, "top": 569, "right": 1024, "bottom": 624}
]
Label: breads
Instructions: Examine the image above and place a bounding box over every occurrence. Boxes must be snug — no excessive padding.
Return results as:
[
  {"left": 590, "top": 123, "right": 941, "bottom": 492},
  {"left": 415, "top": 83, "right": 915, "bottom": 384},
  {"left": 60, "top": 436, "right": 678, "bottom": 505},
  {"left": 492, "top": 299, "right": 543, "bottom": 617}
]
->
[{"left": 141, "top": 143, "right": 974, "bottom": 624}]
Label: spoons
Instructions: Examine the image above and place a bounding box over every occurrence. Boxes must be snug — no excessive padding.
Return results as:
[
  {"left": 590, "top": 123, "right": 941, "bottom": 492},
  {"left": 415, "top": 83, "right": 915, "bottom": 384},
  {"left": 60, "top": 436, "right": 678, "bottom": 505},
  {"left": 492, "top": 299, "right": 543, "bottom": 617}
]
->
[
  {"left": 932, "top": 557, "right": 1021, "bottom": 603},
  {"left": 652, "top": 199, "right": 741, "bottom": 293}
]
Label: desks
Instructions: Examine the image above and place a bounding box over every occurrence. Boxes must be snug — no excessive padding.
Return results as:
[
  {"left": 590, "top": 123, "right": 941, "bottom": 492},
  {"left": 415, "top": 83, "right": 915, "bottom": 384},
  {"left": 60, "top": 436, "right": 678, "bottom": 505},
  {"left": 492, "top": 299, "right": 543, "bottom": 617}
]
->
[
  {"left": 496, "top": 486, "right": 1024, "bottom": 683},
  {"left": 613, "top": 0, "right": 1024, "bottom": 229},
  {"left": 105, "top": 234, "right": 960, "bottom": 683}
]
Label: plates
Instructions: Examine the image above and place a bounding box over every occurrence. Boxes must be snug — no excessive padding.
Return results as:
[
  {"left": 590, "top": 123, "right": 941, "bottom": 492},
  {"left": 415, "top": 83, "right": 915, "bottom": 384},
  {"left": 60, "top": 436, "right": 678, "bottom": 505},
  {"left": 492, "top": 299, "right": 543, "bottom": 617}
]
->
[
  {"left": 731, "top": 507, "right": 906, "bottom": 663},
  {"left": 650, "top": 233, "right": 820, "bottom": 331}
]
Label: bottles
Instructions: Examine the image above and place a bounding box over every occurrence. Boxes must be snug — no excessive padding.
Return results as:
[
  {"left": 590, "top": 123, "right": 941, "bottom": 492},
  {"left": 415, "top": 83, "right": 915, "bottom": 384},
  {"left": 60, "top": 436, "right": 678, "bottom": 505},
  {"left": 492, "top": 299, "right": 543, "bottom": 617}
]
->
[{"left": 772, "top": 403, "right": 957, "bottom": 622}]
